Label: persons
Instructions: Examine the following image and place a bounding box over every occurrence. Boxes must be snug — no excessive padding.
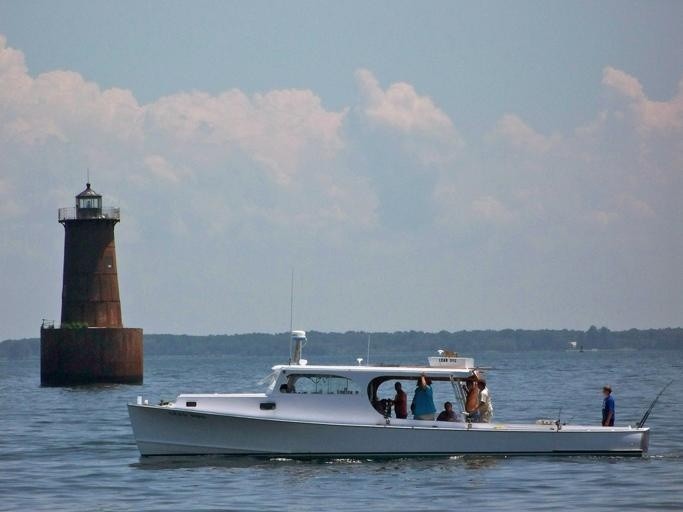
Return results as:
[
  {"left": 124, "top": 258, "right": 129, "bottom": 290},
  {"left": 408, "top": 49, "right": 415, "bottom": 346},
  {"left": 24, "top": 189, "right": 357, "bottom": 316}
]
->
[
  {"left": 461, "top": 380, "right": 480, "bottom": 423},
  {"left": 599, "top": 385, "right": 615, "bottom": 427},
  {"left": 458, "top": 369, "right": 493, "bottom": 424},
  {"left": 386, "top": 381, "right": 408, "bottom": 419},
  {"left": 436, "top": 401, "right": 457, "bottom": 421},
  {"left": 409, "top": 375, "right": 435, "bottom": 420}
]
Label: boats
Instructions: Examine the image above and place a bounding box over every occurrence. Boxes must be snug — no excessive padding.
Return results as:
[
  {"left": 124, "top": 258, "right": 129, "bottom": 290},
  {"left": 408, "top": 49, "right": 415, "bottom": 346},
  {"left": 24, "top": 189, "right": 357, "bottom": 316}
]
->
[{"left": 124, "top": 330, "right": 651, "bottom": 458}]
